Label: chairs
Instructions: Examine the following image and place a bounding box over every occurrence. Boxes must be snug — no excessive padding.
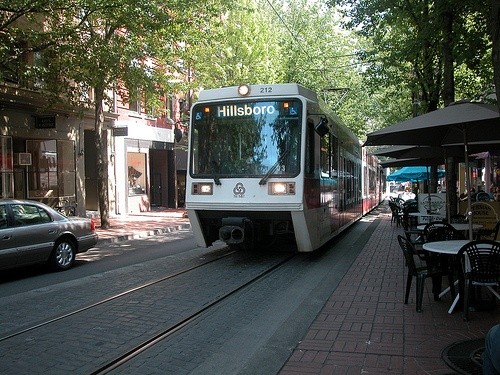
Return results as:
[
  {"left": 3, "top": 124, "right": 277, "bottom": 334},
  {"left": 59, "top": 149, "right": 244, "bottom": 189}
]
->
[{"left": 388, "top": 197, "right": 500, "bottom": 313}]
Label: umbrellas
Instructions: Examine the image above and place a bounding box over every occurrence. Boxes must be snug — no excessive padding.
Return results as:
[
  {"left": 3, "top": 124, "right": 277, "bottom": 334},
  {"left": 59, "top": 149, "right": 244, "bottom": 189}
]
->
[
  {"left": 377, "top": 155, "right": 479, "bottom": 168},
  {"left": 372, "top": 146, "right": 500, "bottom": 159},
  {"left": 359, "top": 101, "right": 500, "bottom": 147},
  {"left": 386, "top": 165, "right": 445, "bottom": 183}
]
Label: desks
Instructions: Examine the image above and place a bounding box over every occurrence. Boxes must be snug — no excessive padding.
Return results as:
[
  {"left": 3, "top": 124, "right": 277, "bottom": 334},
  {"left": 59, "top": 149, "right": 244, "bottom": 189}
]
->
[
  {"left": 417, "top": 224, "right": 483, "bottom": 231},
  {"left": 398, "top": 212, "right": 442, "bottom": 217},
  {"left": 422, "top": 240, "right": 500, "bottom": 315}
]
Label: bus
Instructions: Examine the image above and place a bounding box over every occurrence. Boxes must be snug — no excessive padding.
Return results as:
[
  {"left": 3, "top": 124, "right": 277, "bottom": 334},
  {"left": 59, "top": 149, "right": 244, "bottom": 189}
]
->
[{"left": 174, "top": 82, "right": 390, "bottom": 254}]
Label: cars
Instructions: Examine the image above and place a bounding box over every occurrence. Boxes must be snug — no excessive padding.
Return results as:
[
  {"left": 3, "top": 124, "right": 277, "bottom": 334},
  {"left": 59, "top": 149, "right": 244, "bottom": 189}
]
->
[{"left": 0, "top": 200, "right": 99, "bottom": 272}]
